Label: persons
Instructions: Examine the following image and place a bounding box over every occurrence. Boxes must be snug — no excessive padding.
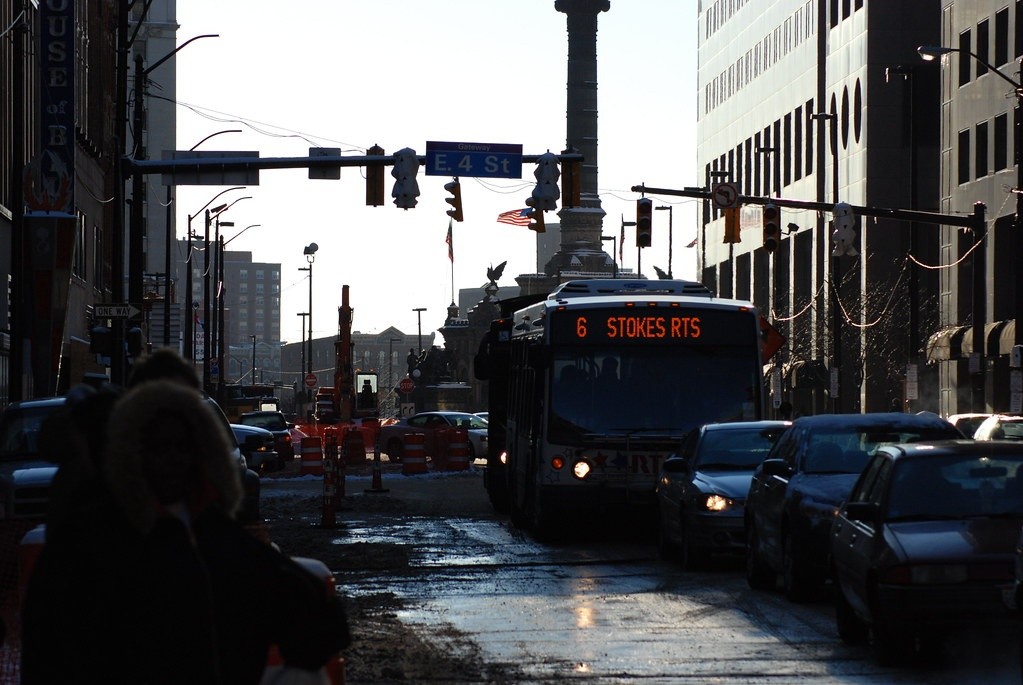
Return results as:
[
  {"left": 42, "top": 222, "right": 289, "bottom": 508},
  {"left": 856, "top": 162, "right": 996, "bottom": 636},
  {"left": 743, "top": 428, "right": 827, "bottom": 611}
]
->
[
  {"left": 888, "top": 398, "right": 904, "bottom": 413},
  {"left": 778, "top": 402, "right": 794, "bottom": 421},
  {"left": 407, "top": 348, "right": 418, "bottom": 377},
  {"left": 0, "top": 347, "right": 353, "bottom": 685}
]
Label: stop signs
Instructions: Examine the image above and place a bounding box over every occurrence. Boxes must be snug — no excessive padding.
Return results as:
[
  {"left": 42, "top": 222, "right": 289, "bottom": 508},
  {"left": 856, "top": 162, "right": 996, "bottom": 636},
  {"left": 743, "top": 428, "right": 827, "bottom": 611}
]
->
[
  {"left": 305, "top": 374, "right": 317, "bottom": 387},
  {"left": 400, "top": 378, "right": 415, "bottom": 393}
]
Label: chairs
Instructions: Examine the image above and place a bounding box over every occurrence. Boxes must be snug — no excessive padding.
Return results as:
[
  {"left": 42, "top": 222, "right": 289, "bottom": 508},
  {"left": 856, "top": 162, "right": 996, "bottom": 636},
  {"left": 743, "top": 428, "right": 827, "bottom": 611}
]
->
[{"left": 805, "top": 442, "right": 843, "bottom": 473}]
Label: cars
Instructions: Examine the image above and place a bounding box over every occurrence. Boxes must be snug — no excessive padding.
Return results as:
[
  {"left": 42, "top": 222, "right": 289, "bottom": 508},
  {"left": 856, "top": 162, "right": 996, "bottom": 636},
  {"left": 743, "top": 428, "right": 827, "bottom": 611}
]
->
[
  {"left": 652, "top": 421, "right": 797, "bottom": 575},
  {"left": 377, "top": 411, "right": 490, "bottom": 466},
  {"left": 228, "top": 423, "right": 280, "bottom": 481},
  {"left": 945, "top": 412, "right": 1023, "bottom": 443},
  {"left": 829, "top": 438, "right": 1023, "bottom": 666},
  {"left": 467, "top": 411, "right": 490, "bottom": 459},
  {"left": 744, "top": 410, "right": 973, "bottom": 606},
  {"left": 238, "top": 410, "right": 297, "bottom": 463}
]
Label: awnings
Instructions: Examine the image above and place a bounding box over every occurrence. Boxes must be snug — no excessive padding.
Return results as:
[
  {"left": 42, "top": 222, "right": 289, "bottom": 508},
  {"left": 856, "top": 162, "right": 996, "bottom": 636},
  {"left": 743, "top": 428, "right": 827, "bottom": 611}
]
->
[
  {"left": 961, "top": 323, "right": 1006, "bottom": 357},
  {"left": 998, "top": 317, "right": 1023, "bottom": 356},
  {"left": 926, "top": 325, "right": 974, "bottom": 362}
]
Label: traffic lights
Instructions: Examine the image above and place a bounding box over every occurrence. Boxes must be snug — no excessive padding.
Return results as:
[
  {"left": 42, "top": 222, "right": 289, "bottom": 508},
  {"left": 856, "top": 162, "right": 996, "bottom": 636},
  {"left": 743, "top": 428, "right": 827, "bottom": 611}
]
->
[
  {"left": 444, "top": 181, "right": 460, "bottom": 221},
  {"left": 636, "top": 199, "right": 651, "bottom": 246},
  {"left": 762, "top": 203, "right": 780, "bottom": 252},
  {"left": 525, "top": 196, "right": 542, "bottom": 231},
  {"left": 390, "top": 149, "right": 416, "bottom": 209}
]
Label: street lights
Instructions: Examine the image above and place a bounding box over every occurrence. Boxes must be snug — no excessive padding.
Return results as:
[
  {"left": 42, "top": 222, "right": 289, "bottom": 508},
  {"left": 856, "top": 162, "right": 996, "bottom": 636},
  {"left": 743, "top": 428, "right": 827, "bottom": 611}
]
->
[
  {"left": 412, "top": 308, "right": 427, "bottom": 356},
  {"left": 389, "top": 338, "right": 402, "bottom": 392},
  {"left": 303, "top": 242, "right": 319, "bottom": 377}
]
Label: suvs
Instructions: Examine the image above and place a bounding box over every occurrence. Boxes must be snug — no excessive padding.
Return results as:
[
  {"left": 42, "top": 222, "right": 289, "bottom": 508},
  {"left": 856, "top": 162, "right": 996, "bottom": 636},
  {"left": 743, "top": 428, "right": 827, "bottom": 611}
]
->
[{"left": 0, "top": 397, "right": 75, "bottom": 522}]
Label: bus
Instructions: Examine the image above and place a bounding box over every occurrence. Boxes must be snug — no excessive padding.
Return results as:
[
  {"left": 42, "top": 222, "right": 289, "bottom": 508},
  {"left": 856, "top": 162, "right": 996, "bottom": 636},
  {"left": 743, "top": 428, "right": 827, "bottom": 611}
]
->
[{"left": 473, "top": 277, "right": 765, "bottom": 542}]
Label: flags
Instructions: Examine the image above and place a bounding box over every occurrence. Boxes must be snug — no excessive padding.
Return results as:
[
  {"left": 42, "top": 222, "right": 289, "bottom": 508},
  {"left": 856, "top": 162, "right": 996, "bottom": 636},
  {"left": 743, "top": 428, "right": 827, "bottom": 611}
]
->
[
  {"left": 446, "top": 226, "right": 453, "bottom": 262},
  {"left": 497, "top": 207, "right": 532, "bottom": 226}
]
